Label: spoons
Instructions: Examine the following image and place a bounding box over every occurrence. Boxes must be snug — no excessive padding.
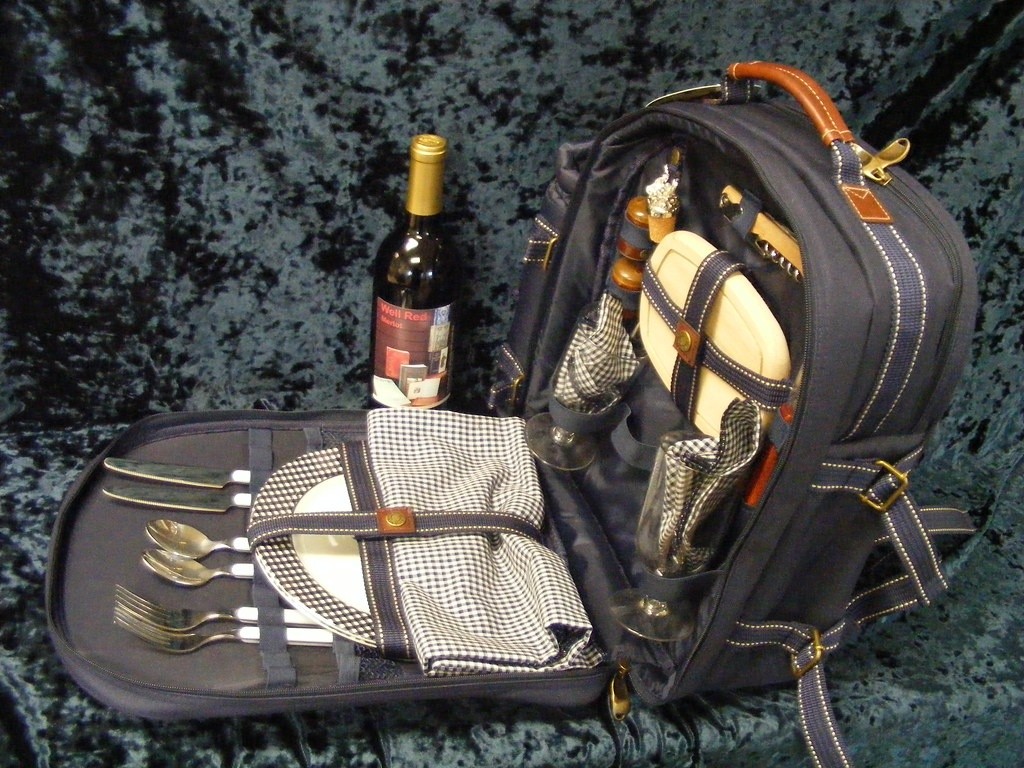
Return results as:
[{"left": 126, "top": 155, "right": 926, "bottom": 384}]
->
[
  {"left": 146, "top": 520, "right": 254, "bottom": 561},
  {"left": 141, "top": 548, "right": 255, "bottom": 587}
]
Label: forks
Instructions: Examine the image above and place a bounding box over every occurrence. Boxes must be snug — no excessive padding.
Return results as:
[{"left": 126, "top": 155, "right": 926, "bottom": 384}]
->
[
  {"left": 113, "top": 604, "right": 333, "bottom": 654},
  {"left": 113, "top": 583, "right": 328, "bottom": 633}
]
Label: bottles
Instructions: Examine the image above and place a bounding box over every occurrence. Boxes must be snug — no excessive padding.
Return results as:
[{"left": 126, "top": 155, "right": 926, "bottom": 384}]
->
[{"left": 365, "top": 134, "right": 453, "bottom": 418}]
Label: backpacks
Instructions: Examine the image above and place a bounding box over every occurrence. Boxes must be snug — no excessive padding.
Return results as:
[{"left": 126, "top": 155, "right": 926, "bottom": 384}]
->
[{"left": 42, "top": 60, "right": 978, "bottom": 768}]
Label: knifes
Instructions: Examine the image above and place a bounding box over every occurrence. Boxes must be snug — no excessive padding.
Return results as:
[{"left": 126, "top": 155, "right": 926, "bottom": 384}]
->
[
  {"left": 101, "top": 487, "right": 254, "bottom": 513},
  {"left": 104, "top": 457, "right": 272, "bottom": 489}
]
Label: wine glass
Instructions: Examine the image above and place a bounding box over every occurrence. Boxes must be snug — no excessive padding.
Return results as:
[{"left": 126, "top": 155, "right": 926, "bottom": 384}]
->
[
  {"left": 524, "top": 300, "right": 647, "bottom": 471},
  {"left": 605, "top": 426, "right": 740, "bottom": 642}
]
